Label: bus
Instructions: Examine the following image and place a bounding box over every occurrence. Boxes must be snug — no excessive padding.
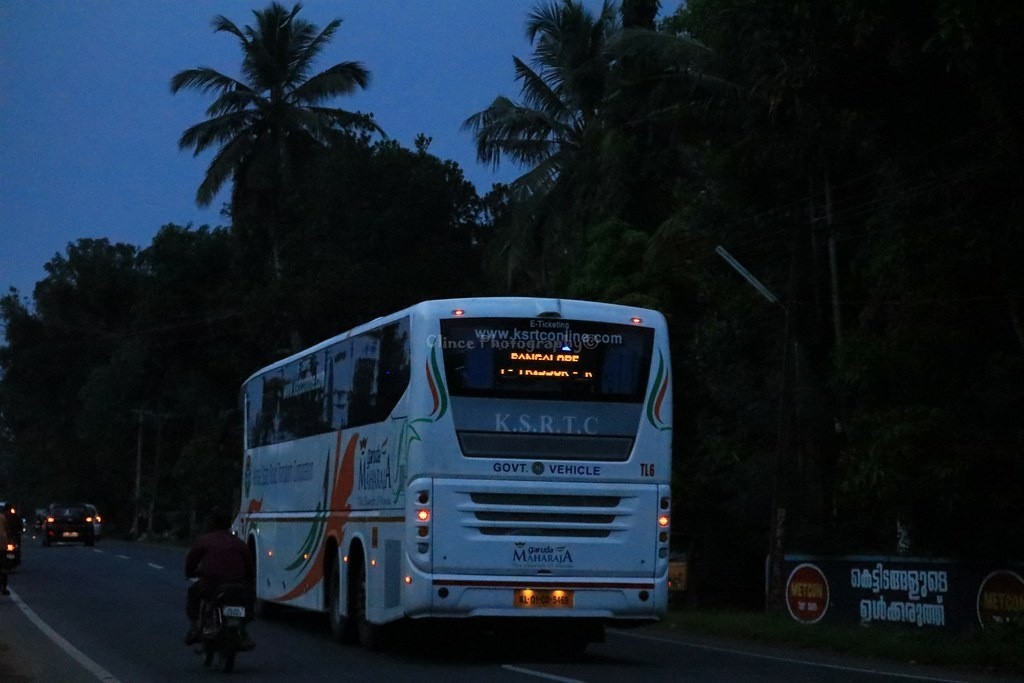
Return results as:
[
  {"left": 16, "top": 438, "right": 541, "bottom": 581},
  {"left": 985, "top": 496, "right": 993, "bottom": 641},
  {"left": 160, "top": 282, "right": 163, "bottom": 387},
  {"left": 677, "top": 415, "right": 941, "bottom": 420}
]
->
[{"left": 232, "top": 295, "right": 674, "bottom": 657}]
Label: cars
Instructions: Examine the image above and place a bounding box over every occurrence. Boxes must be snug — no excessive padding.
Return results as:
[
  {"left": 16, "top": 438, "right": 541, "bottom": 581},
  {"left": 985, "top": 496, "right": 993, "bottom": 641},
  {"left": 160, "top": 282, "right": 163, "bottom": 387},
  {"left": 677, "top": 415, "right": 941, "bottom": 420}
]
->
[
  {"left": 0, "top": 502, "right": 23, "bottom": 568},
  {"left": 42, "top": 503, "right": 95, "bottom": 547},
  {"left": 85, "top": 504, "right": 102, "bottom": 542}
]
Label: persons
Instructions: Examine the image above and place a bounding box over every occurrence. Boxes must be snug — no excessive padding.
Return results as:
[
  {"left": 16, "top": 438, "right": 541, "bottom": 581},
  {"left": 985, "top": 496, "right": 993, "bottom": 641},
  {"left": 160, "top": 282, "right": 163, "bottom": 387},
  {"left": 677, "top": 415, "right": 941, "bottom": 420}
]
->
[{"left": 184, "top": 506, "right": 257, "bottom": 646}]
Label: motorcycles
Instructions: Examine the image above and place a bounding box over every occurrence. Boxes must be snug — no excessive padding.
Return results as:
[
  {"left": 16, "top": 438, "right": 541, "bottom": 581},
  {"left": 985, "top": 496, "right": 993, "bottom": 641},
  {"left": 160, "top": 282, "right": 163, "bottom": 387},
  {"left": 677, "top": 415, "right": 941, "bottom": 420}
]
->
[{"left": 185, "top": 571, "right": 253, "bottom": 676}]
